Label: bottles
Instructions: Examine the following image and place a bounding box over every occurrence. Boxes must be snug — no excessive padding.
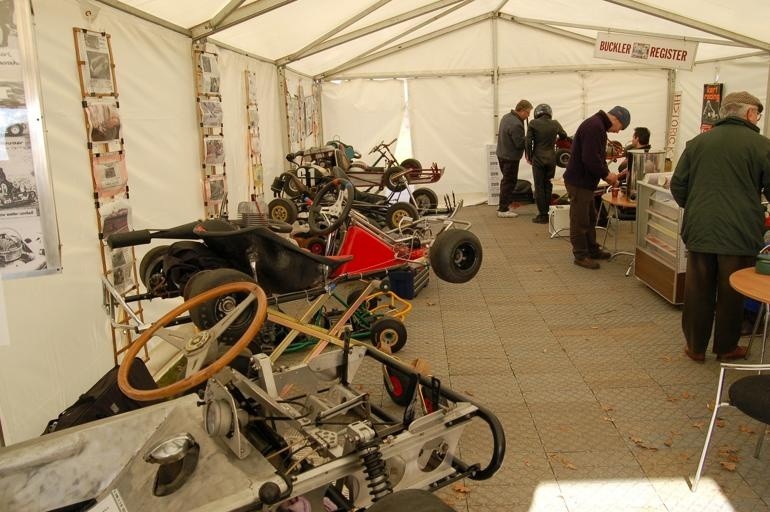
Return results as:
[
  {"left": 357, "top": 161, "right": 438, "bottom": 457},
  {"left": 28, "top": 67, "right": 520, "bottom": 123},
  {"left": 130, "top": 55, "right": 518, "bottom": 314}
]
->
[
  {"left": 608, "top": 106, "right": 630, "bottom": 130},
  {"left": 722, "top": 91, "right": 763, "bottom": 114}
]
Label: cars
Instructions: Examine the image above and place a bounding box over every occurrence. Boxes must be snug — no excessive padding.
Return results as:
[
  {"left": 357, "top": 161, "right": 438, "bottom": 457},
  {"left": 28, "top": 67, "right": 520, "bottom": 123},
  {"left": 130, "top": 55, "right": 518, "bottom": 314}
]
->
[{"left": 612, "top": 187, "right": 619, "bottom": 196}]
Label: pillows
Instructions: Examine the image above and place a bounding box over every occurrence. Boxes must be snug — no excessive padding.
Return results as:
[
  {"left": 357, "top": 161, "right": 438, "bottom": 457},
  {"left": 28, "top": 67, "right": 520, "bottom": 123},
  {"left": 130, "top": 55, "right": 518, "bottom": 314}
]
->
[{"left": 534, "top": 104, "right": 552, "bottom": 119}]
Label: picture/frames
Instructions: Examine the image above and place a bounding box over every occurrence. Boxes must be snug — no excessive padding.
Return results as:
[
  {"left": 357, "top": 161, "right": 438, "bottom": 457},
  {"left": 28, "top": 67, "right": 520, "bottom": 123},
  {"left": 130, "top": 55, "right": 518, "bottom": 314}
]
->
[
  {"left": 731, "top": 266, "right": 770, "bottom": 372},
  {"left": 598, "top": 190, "right": 637, "bottom": 253}
]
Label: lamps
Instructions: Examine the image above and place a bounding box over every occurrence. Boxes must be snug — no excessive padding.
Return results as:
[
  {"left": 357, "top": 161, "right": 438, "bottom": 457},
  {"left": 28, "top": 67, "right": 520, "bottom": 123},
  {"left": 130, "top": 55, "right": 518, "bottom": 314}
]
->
[
  {"left": 690, "top": 361, "right": 770, "bottom": 494},
  {"left": 191, "top": 216, "right": 354, "bottom": 294}
]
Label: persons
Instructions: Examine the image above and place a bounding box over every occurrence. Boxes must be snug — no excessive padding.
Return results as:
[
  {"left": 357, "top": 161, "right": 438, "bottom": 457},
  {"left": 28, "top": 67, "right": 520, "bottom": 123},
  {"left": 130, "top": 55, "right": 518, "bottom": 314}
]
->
[
  {"left": 617, "top": 126, "right": 652, "bottom": 183},
  {"left": 668, "top": 91, "right": 769, "bottom": 362},
  {"left": 562, "top": 106, "right": 631, "bottom": 272},
  {"left": 493, "top": 99, "right": 533, "bottom": 218},
  {"left": 524, "top": 103, "right": 568, "bottom": 226}
]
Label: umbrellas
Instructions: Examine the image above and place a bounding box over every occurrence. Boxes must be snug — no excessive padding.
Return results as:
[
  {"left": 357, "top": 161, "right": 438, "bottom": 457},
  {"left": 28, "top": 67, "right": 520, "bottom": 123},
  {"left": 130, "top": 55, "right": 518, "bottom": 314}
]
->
[
  {"left": 685, "top": 345, "right": 705, "bottom": 363},
  {"left": 532, "top": 218, "right": 548, "bottom": 224},
  {"left": 717, "top": 346, "right": 748, "bottom": 362}
]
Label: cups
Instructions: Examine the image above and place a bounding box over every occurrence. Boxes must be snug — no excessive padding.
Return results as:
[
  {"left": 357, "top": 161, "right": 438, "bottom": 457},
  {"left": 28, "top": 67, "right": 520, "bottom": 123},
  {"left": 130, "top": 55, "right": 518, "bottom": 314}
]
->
[{"left": 756, "top": 110, "right": 762, "bottom": 120}]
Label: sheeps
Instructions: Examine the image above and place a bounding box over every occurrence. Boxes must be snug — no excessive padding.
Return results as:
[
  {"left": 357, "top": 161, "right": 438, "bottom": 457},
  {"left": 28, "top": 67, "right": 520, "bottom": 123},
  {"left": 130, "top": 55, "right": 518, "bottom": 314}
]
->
[{"left": 614, "top": 180, "right": 628, "bottom": 187}]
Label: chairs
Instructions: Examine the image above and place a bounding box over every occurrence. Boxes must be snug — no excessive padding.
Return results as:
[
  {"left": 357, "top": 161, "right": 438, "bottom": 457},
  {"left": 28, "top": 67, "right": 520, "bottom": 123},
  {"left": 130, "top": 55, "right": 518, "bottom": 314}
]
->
[
  {"left": 496, "top": 206, "right": 518, "bottom": 218},
  {"left": 574, "top": 256, "right": 600, "bottom": 269},
  {"left": 591, "top": 251, "right": 611, "bottom": 260}
]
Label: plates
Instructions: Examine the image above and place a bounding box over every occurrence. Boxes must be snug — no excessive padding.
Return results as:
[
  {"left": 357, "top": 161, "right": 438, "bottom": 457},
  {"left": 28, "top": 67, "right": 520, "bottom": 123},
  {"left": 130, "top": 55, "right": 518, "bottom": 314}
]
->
[{"left": 635, "top": 180, "right": 689, "bottom": 306}]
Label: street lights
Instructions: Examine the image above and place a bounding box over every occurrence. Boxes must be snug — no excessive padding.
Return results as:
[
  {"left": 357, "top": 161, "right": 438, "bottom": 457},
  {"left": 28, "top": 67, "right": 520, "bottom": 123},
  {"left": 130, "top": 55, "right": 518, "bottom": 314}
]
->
[{"left": 756, "top": 254, "right": 770, "bottom": 275}]
